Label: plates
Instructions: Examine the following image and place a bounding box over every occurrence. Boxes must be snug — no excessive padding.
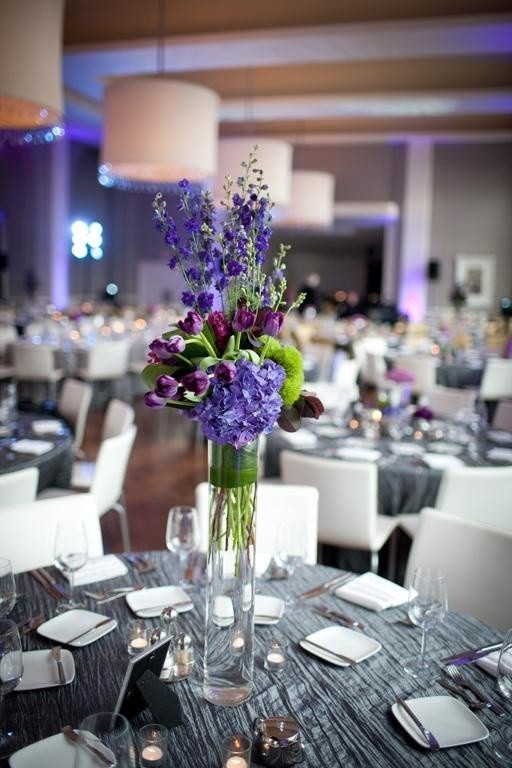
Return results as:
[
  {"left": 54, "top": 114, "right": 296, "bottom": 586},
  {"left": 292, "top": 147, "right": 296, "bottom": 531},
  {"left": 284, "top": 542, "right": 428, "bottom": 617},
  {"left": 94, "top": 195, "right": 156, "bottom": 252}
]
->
[
  {"left": 37, "top": 609, "right": 119, "bottom": 648},
  {"left": 126, "top": 584, "right": 195, "bottom": 619},
  {"left": 391, "top": 695, "right": 490, "bottom": 751},
  {"left": 299, "top": 626, "right": 382, "bottom": 667},
  {"left": 254, "top": 594, "right": 285, "bottom": 628},
  {"left": 10, "top": 729, "right": 119, "bottom": 768},
  {"left": 14, "top": 648, "right": 75, "bottom": 690},
  {"left": 281, "top": 416, "right": 512, "bottom": 468}
]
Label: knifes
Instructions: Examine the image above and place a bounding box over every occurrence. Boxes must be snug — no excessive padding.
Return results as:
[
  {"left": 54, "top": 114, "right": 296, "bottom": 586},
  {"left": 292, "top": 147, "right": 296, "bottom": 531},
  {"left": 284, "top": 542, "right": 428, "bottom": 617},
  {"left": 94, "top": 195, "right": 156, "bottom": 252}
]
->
[
  {"left": 396, "top": 696, "right": 441, "bottom": 752},
  {"left": 57, "top": 723, "right": 117, "bottom": 768},
  {"left": 133, "top": 600, "right": 193, "bottom": 616},
  {"left": 53, "top": 646, "right": 69, "bottom": 686},
  {"left": 442, "top": 642, "right": 506, "bottom": 665},
  {"left": 255, "top": 612, "right": 281, "bottom": 620},
  {"left": 300, "top": 635, "right": 359, "bottom": 666},
  {"left": 62, "top": 618, "right": 115, "bottom": 646},
  {"left": 297, "top": 571, "right": 352, "bottom": 600}
]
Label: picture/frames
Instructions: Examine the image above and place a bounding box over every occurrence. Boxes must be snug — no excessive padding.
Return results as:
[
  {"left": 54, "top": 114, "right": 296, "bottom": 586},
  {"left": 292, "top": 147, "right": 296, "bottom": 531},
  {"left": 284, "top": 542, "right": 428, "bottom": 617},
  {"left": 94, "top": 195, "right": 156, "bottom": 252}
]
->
[{"left": 455, "top": 256, "right": 494, "bottom": 307}]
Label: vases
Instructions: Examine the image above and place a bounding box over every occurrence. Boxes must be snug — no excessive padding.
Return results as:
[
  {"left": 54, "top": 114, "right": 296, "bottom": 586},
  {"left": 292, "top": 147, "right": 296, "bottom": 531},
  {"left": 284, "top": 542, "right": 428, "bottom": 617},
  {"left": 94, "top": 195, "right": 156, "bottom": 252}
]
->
[{"left": 202, "top": 437, "right": 258, "bottom": 708}]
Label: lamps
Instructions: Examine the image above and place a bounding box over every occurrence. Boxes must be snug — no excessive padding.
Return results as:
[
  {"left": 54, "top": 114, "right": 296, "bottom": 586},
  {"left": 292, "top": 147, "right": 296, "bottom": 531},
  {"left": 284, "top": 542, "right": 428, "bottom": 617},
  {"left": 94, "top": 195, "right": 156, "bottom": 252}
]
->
[
  {"left": 271, "top": 169, "right": 336, "bottom": 228},
  {"left": 97, "top": 0, "right": 220, "bottom": 196},
  {"left": 213, "top": 136, "right": 294, "bottom": 208},
  {"left": 0, "top": 0, "right": 67, "bottom": 146}
]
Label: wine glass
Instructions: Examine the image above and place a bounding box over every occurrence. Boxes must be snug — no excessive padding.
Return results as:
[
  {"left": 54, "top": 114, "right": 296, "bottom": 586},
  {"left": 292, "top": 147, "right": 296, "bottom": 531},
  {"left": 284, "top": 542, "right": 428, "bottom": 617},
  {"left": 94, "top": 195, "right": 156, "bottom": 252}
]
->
[
  {"left": 0, "top": 557, "right": 18, "bottom": 617},
  {"left": 2, "top": 619, "right": 24, "bottom": 744},
  {"left": 166, "top": 507, "right": 199, "bottom": 589},
  {"left": 403, "top": 570, "right": 448, "bottom": 681},
  {"left": 54, "top": 520, "right": 88, "bottom": 614},
  {"left": 493, "top": 629, "right": 511, "bottom": 761}
]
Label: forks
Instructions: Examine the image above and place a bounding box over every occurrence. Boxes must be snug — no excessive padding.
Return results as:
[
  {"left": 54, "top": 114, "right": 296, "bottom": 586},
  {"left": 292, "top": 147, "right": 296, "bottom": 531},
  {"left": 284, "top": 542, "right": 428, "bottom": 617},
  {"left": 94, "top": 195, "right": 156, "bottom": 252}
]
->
[{"left": 446, "top": 664, "right": 506, "bottom": 717}]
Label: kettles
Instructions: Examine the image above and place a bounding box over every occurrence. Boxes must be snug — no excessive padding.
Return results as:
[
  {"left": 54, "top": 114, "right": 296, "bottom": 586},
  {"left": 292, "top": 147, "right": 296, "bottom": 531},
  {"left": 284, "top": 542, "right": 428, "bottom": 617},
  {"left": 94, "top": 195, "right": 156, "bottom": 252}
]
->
[{"left": 249, "top": 716, "right": 308, "bottom": 768}]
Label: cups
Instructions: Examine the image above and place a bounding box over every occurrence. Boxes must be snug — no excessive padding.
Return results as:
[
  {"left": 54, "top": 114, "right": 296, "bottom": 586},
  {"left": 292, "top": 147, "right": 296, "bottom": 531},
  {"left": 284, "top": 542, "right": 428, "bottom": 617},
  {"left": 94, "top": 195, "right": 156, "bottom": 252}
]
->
[
  {"left": 73, "top": 712, "right": 137, "bottom": 767},
  {"left": 219, "top": 734, "right": 252, "bottom": 768},
  {"left": 126, "top": 621, "right": 151, "bottom": 656},
  {"left": 173, "top": 644, "right": 194, "bottom": 677},
  {"left": 137, "top": 724, "right": 169, "bottom": 767},
  {"left": 265, "top": 637, "right": 287, "bottom": 672}
]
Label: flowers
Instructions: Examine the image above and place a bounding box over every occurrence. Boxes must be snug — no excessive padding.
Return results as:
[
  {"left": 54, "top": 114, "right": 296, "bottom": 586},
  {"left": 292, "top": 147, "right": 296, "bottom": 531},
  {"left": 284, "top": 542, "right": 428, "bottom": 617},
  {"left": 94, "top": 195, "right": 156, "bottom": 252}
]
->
[{"left": 143, "top": 144, "right": 324, "bottom": 586}]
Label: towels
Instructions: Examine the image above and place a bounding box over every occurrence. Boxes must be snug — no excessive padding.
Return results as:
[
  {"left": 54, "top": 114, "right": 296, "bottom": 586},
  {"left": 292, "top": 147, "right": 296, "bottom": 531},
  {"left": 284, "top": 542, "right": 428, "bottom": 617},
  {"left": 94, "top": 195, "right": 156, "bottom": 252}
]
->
[{"left": 477, "top": 649, "right": 512, "bottom": 677}]
